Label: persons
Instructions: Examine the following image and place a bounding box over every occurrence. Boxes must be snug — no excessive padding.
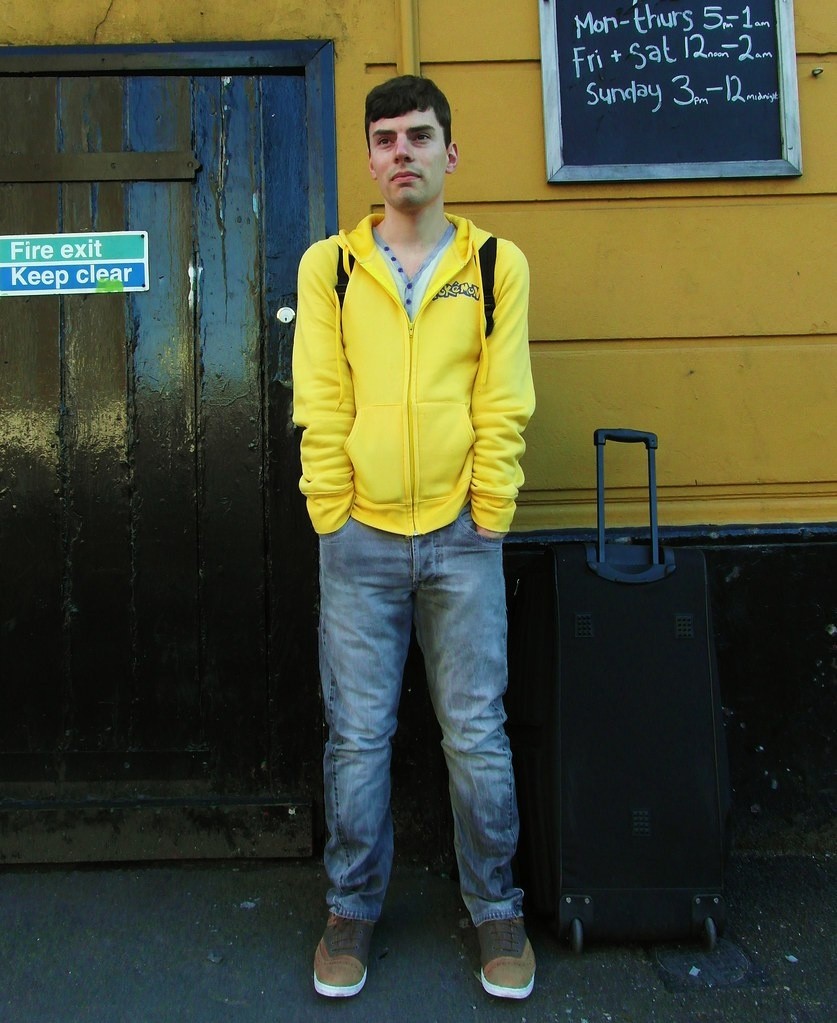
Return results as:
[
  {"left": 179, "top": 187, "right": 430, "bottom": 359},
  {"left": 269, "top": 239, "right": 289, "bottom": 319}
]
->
[{"left": 290, "top": 72, "right": 536, "bottom": 1002}]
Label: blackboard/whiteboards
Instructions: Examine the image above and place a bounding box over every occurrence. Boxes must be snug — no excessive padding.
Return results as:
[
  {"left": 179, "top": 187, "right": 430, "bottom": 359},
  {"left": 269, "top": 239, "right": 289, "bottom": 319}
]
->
[{"left": 536, "top": 0, "right": 802, "bottom": 183}]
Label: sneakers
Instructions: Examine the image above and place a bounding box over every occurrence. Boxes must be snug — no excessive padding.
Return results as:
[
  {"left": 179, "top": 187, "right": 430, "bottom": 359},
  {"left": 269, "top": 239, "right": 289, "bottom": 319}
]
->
[
  {"left": 313, "top": 913, "right": 373, "bottom": 997},
  {"left": 477, "top": 913, "right": 536, "bottom": 998}
]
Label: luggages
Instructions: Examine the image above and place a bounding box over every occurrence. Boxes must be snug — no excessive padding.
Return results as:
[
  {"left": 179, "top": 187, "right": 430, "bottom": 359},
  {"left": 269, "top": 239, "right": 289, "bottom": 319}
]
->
[{"left": 526, "top": 428, "right": 729, "bottom": 954}]
